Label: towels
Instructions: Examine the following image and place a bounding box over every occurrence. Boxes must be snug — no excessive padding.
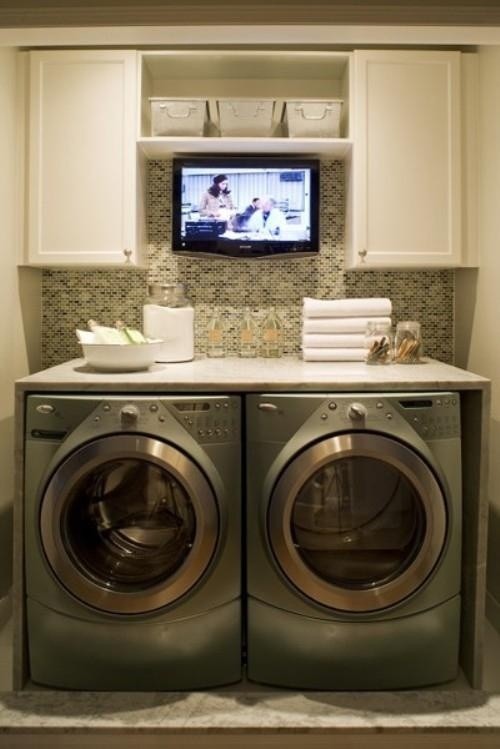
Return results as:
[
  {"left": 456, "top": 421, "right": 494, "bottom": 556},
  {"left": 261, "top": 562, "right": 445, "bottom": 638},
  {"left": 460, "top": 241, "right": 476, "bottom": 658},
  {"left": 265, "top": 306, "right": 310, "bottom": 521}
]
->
[{"left": 302, "top": 296, "right": 393, "bottom": 362}]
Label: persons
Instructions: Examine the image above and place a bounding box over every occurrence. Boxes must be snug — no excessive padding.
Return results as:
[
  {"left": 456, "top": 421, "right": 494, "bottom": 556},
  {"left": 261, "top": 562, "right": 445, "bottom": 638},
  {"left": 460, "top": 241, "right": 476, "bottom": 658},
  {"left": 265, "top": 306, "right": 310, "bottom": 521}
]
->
[
  {"left": 199, "top": 175, "right": 236, "bottom": 232},
  {"left": 242, "top": 199, "right": 287, "bottom": 234},
  {"left": 236, "top": 198, "right": 261, "bottom": 232}
]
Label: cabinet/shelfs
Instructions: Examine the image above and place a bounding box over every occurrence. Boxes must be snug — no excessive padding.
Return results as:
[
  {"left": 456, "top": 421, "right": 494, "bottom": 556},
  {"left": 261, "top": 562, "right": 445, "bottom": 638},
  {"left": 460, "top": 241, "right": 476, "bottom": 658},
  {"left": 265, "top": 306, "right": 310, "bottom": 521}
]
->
[
  {"left": 16, "top": 46, "right": 145, "bottom": 271},
  {"left": 343, "top": 49, "right": 480, "bottom": 271}
]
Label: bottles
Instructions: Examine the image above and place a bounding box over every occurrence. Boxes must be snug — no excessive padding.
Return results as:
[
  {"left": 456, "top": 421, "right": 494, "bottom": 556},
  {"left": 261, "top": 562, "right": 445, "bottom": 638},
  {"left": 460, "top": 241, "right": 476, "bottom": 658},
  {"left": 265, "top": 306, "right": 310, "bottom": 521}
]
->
[
  {"left": 141, "top": 284, "right": 194, "bottom": 363},
  {"left": 259, "top": 307, "right": 285, "bottom": 357},
  {"left": 394, "top": 321, "right": 423, "bottom": 363},
  {"left": 366, "top": 321, "right": 391, "bottom": 365},
  {"left": 236, "top": 307, "right": 259, "bottom": 357},
  {"left": 205, "top": 310, "right": 226, "bottom": 358}
]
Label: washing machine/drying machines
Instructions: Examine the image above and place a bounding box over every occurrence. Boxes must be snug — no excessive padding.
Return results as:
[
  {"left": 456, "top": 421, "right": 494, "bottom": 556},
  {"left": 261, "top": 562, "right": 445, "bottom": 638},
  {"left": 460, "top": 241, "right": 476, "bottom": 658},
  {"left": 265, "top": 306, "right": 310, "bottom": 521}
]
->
[
  {"left": 23, "top": 394, "right": 242, "bottom": 691},
  {"left": 245, "top": 393, "right": 462, "bottom": 691}
]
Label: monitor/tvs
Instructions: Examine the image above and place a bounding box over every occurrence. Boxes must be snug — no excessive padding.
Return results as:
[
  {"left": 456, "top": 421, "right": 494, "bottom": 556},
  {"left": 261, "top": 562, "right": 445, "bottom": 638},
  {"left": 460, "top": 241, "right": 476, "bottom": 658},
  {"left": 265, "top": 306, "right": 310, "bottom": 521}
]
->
[{"left": 170, "top": 155, "right": 322, "bottom": 259}]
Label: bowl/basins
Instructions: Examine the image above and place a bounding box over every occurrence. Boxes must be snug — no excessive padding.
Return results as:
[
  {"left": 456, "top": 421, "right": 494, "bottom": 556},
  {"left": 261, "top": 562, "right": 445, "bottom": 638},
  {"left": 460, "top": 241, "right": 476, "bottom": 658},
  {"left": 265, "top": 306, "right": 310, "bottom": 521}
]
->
[{"left": 78, "top": 339, "right": 165, "bottom": 372}]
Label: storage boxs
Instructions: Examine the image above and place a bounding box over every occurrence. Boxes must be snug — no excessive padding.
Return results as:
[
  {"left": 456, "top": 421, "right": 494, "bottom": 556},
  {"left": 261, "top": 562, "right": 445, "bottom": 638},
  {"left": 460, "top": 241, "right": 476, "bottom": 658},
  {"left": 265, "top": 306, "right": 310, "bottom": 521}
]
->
[
  {"left": 206, "top": 98, "right": 281, "bottom": 136},
  {"left": 281, "top": 99, "right": 345, "bottom": 138},
  {"left": 148, "top": 95, "right": 206, "bottom": 137}
]
